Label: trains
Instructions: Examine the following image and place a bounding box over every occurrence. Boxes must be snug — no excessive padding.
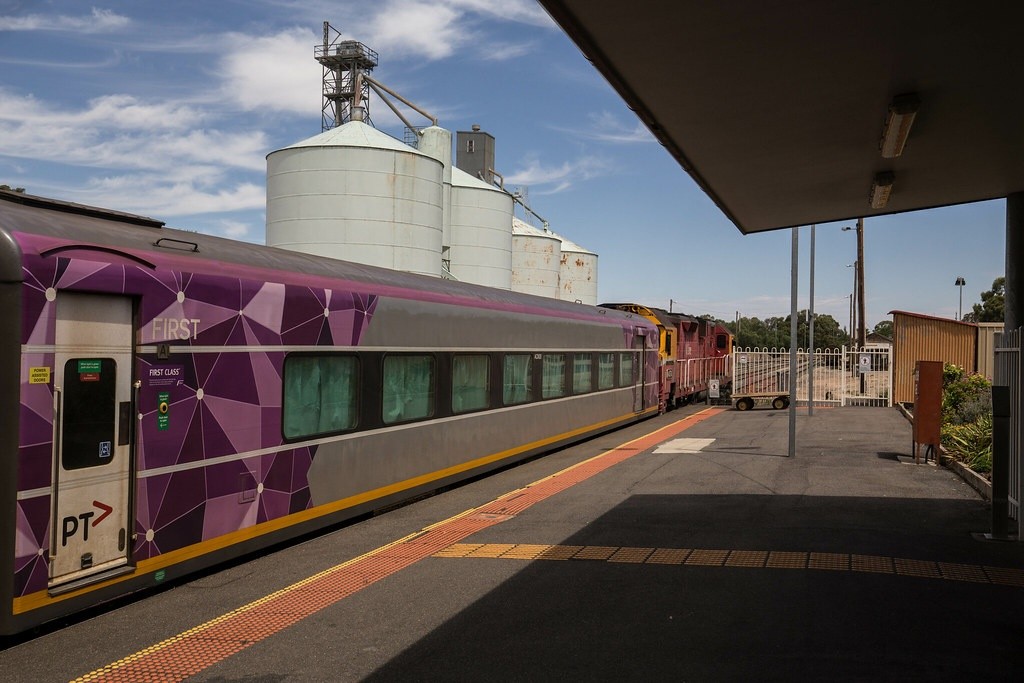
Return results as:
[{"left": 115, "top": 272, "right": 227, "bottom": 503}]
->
[{"left": 0, "top": 188, "right": 737, "bottom": 637}]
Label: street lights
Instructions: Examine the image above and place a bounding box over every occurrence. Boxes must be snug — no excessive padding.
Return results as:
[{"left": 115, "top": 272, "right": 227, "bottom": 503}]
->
[
  {"left": 844, "top": 293, "right": 852, "bottom": 372},
  {"left": 841, "top": 217, "right": 866, "bottom": 395},
  {"left": 845, "top": 260, "right": 858, "bottom": 377}
]
